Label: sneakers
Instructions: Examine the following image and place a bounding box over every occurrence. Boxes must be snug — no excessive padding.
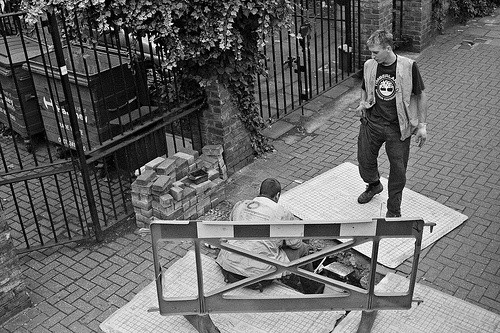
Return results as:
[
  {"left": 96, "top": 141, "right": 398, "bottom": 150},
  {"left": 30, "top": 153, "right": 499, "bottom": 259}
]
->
[
  {"left": 386, "top": 210, "right": 401, "bottom": 218},
  {"left": 358, "top": 183, "right": 384, "bottom": 204}
]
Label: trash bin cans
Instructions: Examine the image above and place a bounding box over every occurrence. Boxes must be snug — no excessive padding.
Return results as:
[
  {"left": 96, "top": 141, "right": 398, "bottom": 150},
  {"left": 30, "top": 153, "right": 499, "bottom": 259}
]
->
[
  {"left": 0, "top": 36, "right": 55, "bottom": 153},
  {"left": 22, "top": 45, "right": 152, "bottom": 177}
]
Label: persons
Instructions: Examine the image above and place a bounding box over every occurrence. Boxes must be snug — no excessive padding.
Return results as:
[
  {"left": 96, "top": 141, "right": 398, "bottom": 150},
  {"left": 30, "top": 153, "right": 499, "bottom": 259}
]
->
[
  {"left": 352, "top": 30, "right": 427, "bottom": 218},
  {"left": 215, "top": 177, "right": 326, "bottom": 295}
]
